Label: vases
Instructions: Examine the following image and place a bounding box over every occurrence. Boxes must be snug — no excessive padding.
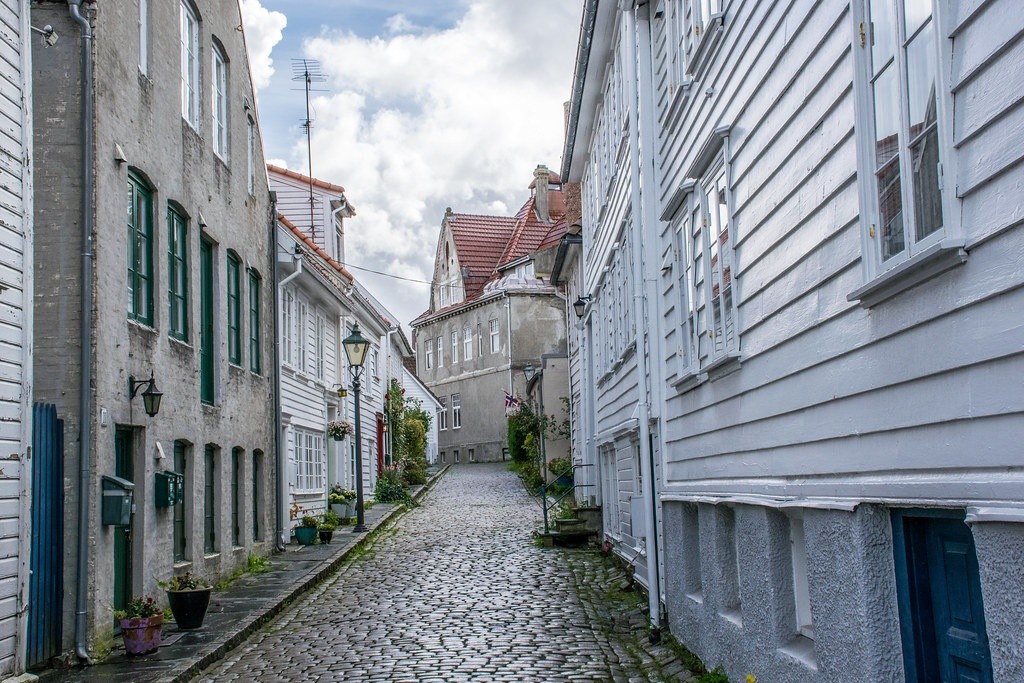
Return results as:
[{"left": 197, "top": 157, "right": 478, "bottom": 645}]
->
[
  {"left": 120, "top": 612, "right": 165, "bottom": 656},
  {"left": 331, "top": 504, "right": 346, "bottom": 518},
  {"left": 334, "top": 432, "right": 345, "bottom": 441},
  {"left": 346, "top": 499, "right": 356, "bottom": 516},
  {"left": 294, "top": 514, "right": 320, "bottom": 545},
  {"left": 165, "top": 584, "right": 213, "bottom": 630},
  {"left": 316, "top": 523, "right": 335, "bottom": 544}
]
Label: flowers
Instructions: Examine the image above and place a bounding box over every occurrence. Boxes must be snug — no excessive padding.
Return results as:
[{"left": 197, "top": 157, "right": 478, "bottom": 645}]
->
[
  {"left": 328, "top": 494, "right": 350, "bottom": 506},
  {"left": 327, "top": 419, "right": 354, "bottom": 438},
  {"left": 109, "top": 594, "right": 161, "bottom": 620},
  {"left": 153, "top": 571, "right": 209, "bottom": 591},
  {"left": 329, "top": 482, "right": 356, "bottom": 501}
]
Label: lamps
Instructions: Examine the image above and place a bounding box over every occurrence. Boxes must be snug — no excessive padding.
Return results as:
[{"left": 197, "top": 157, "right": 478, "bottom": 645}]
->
[
  {"left": 332, "top": 383, "right": 347, "bottom": 397},
  {"left": 573, "top": 293, "right": 592, "bottom": 321},
  {"left": 128, "top": 370, "right": 164, "bottom": 418}
]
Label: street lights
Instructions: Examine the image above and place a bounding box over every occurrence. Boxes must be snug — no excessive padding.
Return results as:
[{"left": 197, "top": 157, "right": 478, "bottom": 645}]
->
[{"left": 342, "top": 320, "right": 372, "bottom": 533}]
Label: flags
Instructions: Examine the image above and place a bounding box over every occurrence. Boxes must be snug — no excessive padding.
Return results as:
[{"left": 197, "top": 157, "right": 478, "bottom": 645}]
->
[{"left": 505, "top": 395, "right": 522, "bottom": 417}]
